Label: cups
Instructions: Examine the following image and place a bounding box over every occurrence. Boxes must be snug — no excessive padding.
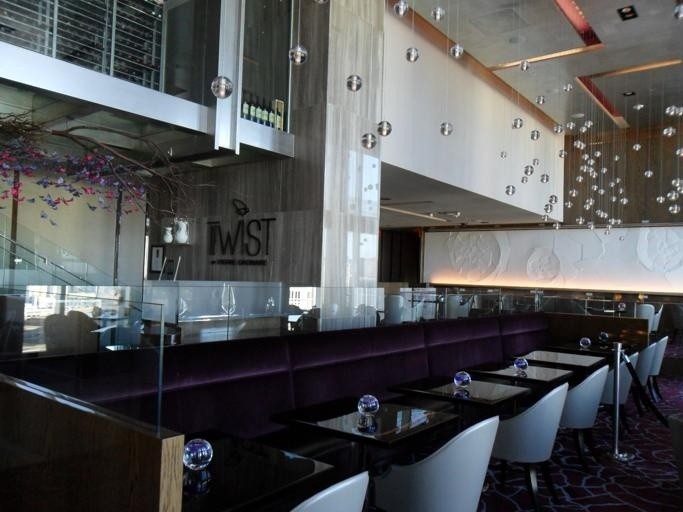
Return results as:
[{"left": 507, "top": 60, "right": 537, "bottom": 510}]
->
[
  {"left": 384, "top": 295, "right": 404, "bottom": 326},
  {"left": 446, "top": 295, "right": 460, "bottom": 319}
]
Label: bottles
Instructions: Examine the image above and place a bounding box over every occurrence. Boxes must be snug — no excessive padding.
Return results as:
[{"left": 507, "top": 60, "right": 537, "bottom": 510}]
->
[
  {"left": 163, "top": 226, "right": 174, "bottom": 244},
  {"left": 174, "top": 220, "right": 188, "bottom": 244},
  {"left": 241, "top": 89, "right": 281, "bottom": 130}
]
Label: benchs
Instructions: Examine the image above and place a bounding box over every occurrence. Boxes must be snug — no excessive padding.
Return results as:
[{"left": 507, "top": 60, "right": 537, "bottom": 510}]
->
[{"left": 40, "top": 312, "right": 552, "bottom": 460}]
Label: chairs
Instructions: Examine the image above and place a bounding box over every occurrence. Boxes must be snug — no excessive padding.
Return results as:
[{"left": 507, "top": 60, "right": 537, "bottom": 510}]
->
[
  {"left": 492, "top": 382, "right": 568, "bottom": 510},
  {"left": 371, "top": 416, "right": 499, "bottom": 512},
  {"left": 38, "top": 285, "right": 671, "bottom": 352},
  {"left": 291, "top": 471, "right": 370, "bottom": 512},
  {"left": 558, "top": 336, "right": 671, "bottom": 466}
]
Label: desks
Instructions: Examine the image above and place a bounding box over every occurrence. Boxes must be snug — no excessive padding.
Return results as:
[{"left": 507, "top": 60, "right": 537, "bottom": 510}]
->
[
  {"left": 489, "top": 349, "right": 606, "bottom": 386},
  {"left": 316, "top": 403, "right": 446, "bottom": 461},
  {"left": 174, "top": 425, "right": 335, "bottom": 512},
  {"left": 432, "top": 378, "right": 530, "bottom": 426}
]
